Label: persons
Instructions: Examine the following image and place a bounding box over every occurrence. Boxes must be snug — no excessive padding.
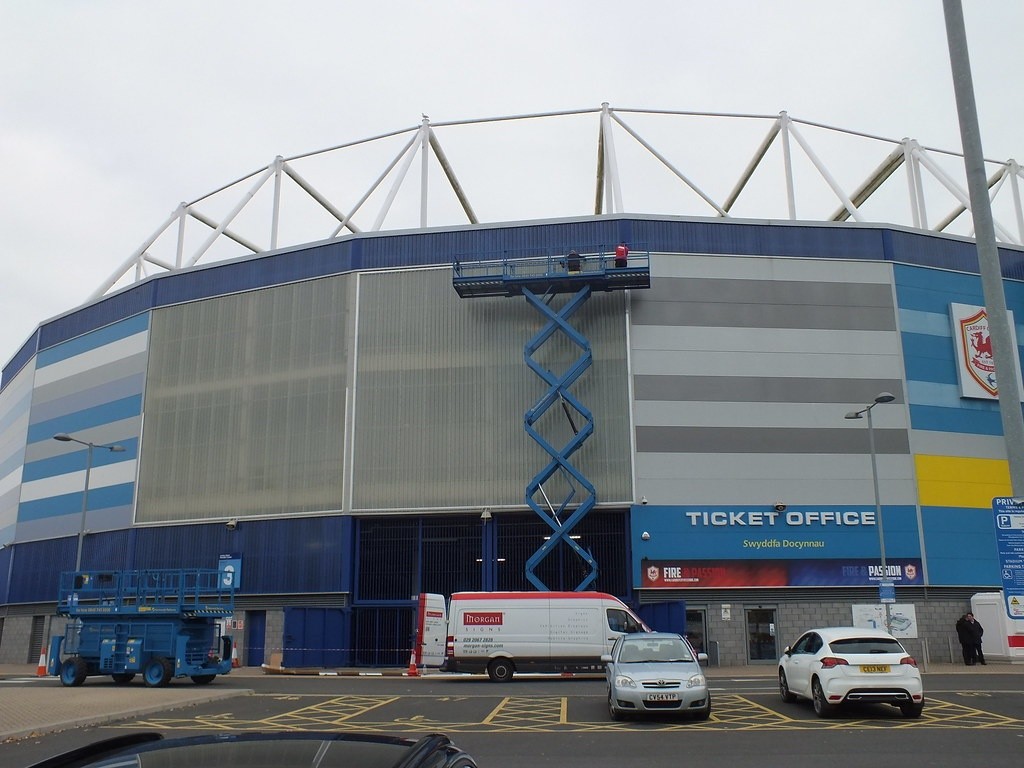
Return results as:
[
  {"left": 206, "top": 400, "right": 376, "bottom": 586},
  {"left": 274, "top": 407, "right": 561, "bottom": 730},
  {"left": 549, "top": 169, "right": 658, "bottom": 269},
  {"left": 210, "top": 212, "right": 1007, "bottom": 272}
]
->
[
  {"left": 562, "top": 249, "right": 587, "bottom": 275},
  {"left": 615, "top": 241, "right": 630, "bottom": 268},
  {"left": 956, "top": 612, "right": 987, "bottom": 666}
]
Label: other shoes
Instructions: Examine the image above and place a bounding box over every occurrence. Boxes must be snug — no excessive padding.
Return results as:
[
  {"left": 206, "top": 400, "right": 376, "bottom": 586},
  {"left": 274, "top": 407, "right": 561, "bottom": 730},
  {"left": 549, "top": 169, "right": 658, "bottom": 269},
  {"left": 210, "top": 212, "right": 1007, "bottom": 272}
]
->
[{"left": 982, "top": 663, "right": 987, "bottom": 665}]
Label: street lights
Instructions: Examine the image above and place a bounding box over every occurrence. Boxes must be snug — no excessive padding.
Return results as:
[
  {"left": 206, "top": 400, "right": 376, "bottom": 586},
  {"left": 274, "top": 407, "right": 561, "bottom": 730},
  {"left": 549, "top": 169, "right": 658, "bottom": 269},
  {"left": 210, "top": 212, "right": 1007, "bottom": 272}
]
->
[
  {"left": 843, "top": 391, "right": 898, "bottom": 636},
  {"left": 51, "top": 433, "right": 127, "bottom": 656}
]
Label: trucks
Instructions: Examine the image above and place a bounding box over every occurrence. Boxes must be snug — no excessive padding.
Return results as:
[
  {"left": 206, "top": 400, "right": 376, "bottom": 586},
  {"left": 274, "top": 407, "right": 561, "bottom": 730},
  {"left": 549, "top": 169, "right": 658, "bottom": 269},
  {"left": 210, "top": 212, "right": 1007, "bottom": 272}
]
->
[
  {"left": 414, "top": 591, "right": 673, "bottom": 684},
  {"left": 48, "top": 569, "right": 236, "bottom": 688}
]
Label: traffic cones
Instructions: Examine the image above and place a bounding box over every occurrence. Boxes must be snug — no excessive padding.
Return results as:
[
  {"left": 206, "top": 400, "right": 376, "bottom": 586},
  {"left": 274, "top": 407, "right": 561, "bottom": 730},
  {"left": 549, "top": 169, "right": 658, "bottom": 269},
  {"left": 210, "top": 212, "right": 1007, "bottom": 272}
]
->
[
  {"left": 231, "top": 641, "right": 240, "bottom": 668},
  {"left": 561, "top": 673, "right": 574, "bottom": 676},
  {"left": 34, "top": 648, "right": 50, "bottom": 676},
  {"left": 407, "top": 650, "right": 420, "bottom": 677}
]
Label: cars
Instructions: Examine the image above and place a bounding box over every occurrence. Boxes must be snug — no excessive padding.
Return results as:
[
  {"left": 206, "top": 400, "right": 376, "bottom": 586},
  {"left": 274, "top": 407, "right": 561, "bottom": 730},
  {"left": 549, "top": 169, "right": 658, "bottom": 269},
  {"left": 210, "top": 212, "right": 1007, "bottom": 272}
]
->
[{"left": 599, "top": 633, "right": 712, "bottom": 722}]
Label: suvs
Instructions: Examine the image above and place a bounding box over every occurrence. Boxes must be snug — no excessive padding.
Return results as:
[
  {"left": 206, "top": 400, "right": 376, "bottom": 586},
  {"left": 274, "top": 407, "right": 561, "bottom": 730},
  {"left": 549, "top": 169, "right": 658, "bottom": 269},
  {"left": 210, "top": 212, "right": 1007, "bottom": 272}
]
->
[
  {"left": 778, "top": 628, "right": 926, "bottom": 720},
  {"left": 25, "top": 730, "right": 480, "bottom": 768}
]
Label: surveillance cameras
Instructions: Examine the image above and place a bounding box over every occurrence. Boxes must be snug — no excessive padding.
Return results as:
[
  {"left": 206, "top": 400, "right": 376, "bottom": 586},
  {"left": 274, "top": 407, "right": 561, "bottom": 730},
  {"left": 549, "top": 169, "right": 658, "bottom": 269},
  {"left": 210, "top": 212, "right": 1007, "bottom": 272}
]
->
[{"left": 642, "top": 499, "right": 647, "bottom": 505}]
